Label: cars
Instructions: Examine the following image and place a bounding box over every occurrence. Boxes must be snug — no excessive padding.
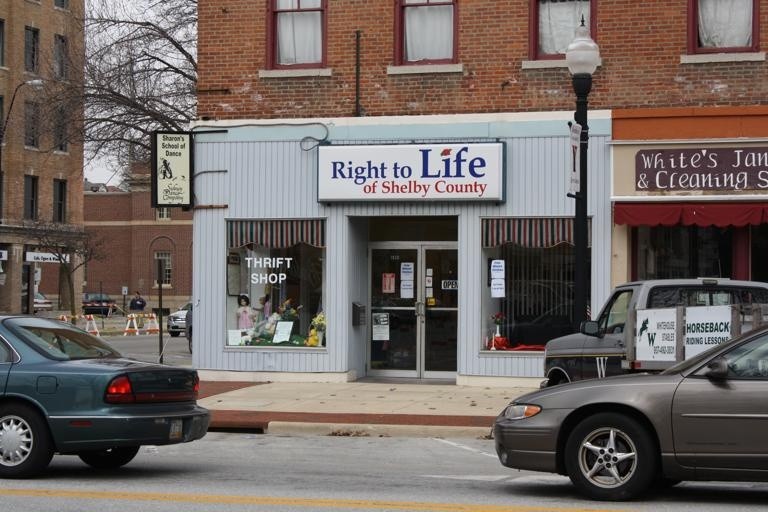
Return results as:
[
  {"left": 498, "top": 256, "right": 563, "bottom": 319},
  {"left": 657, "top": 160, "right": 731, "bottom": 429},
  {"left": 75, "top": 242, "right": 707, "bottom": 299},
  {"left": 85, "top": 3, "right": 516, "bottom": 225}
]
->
[
  {"left": 34, "top": 292, "right": 53, "bottom": 313},
  {"left": 502, "top": 279, "right": 577, "bottom": 324},
  {"left": 85, "top": 292, "right": 117, "bottom": 314},
  {"left": 0, "top": 314, "right": 211, "bottom": 480},
  {"left": 495, "top": 326, "right": 768, "bottom": 502},
  {"left": 167, "top": 301, "right": 192, "bottom": 354}
]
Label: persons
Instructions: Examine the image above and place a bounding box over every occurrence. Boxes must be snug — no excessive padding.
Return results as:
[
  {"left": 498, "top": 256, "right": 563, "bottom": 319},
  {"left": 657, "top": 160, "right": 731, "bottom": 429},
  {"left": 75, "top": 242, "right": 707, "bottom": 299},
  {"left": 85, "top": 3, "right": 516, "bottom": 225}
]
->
[
  {"left": 129, "top": 290, "right": 146, "bottom": 311},
  {"left": 234, "top": 294, "right": 252, "bottom": 331},
  {"left": 251, "top": 294, "right": 267, "bottom": 322}
]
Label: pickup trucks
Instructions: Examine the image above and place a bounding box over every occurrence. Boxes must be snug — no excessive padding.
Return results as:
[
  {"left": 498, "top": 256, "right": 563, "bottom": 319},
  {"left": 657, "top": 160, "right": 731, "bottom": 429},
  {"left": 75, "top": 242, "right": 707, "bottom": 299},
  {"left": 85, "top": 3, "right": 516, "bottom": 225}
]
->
[{"left": 541, "top": 277, "right": 768, "bottom": 388}]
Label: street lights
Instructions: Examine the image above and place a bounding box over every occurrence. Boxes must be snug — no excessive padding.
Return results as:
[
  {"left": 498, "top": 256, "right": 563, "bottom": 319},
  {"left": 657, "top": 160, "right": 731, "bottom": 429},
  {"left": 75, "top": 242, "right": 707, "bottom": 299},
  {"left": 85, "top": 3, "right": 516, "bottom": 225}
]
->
[
  {"left": 0, "top": 79, "right": 48, "bottom": 144},
  {"left": 566, "top": 36, "right": 600, "bottom": 332}
]
